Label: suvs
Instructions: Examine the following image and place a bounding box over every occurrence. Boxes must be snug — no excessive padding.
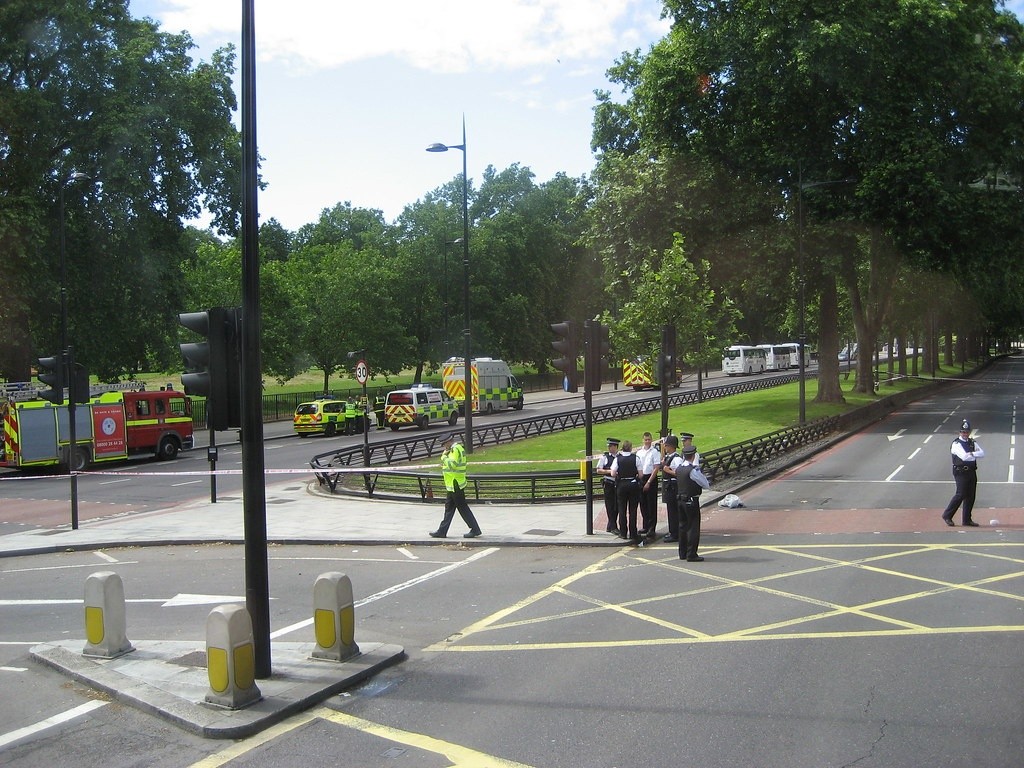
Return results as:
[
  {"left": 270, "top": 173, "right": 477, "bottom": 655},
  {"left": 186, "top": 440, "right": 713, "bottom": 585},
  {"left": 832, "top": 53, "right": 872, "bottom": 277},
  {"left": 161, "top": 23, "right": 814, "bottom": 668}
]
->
[{"left": 293, "top": 394, "right": 373, "bottom": 439}]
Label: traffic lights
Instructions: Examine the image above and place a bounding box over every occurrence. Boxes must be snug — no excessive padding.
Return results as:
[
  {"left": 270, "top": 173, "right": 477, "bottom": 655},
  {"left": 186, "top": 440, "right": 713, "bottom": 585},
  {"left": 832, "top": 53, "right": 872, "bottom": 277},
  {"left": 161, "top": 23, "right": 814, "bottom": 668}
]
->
[
  {"left": 549, "top": 320, "right": 578, "bottom": 393},
  {"left": 656, "top": 352, "right": 676, "bottom": 385},
  {"left": 177, "top": 305, "right": 228, "bottom": 433},
  {"left": 36, "top": 354, "right": 64, "bottom": 406},
  {"left": 582, "top": 319, "right": 609, "bottom": 391}
]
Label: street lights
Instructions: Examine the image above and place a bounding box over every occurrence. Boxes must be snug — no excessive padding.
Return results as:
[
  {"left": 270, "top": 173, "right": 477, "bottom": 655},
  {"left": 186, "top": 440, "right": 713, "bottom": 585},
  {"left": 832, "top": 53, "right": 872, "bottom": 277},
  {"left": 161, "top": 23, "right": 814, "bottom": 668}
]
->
[
  {"left": 54, "top": 147, "right": 91, "bottom": 530},
  {"left": 928, "top": 279, "right": 944, "bottom": 381},
  {"left": 424, "top": 109, "right": 475, "bottom": 455},
  {"left": 791, "top": 161, "right": 857, "bottom": 424}
]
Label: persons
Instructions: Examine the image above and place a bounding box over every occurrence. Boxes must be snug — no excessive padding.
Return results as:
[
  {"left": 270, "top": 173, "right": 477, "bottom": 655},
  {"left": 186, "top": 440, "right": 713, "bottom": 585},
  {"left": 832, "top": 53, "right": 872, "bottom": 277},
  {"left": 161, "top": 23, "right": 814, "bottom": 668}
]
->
[
  {"left": 659, "top": 432, "right": 711, "bottom": 562},
  {"left": 942, "top": 419, "right": 985, "bottom": 526},
  {"left": 338, "top": 390, "right": 386, "bottom": 436},
  {"left": 428, "top": 433, "right": 482, "bottom": 537},
  {"left": 595, "top": 437, "right": 621, "bottom": 534},
  {"left": 636, "top": 432, "right": 661, "bottom": 539},
  {"left": 609, "top": 439, "right": 642, "bottom": 539}
]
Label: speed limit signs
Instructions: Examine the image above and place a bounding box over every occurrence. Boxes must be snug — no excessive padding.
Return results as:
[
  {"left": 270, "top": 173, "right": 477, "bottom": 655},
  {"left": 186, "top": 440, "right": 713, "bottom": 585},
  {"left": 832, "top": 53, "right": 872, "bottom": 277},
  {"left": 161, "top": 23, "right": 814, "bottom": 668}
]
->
[{"left": 355, "top": 361, "right": 369, "bottom": 384}]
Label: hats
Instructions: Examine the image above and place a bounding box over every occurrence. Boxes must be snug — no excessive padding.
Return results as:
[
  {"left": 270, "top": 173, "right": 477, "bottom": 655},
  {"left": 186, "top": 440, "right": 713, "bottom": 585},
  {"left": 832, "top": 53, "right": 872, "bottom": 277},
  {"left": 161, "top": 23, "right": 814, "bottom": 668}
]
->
[
  {"left": 682, "top": 446, "right": 699, "bottom": 454},
  {"left": 680, "top": 433, "right": 694, "bottom": 441},
  {"left": 438, "top": 431, "right": 453, "bottom": 447},
  {"left": 960, "top": 418, "right": 971, "bottom": 433},
  {"left": 663, "top": 436, "right": 680, "bottom": 449},
  {"left": 606, "top": 438, "right": 621, "bottom": 446}
]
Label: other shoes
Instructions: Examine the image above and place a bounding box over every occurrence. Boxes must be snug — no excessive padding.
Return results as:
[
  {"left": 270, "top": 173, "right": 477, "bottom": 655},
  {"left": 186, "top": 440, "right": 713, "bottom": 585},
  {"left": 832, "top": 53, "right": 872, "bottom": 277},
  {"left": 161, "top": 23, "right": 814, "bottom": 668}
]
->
[
  {"left": 943, "top": 518, "right": 954, "bottom": 526},
  {"left": 464, "top": 527, "right": 481, "bottom": 538},
  {"left": 962, "top": 521, "right": 979, "bottom": 527},
  {"left": 680, "top": 554, "right": 704, "bottom": 562},
  {"left": 618, "top": 534, "right": 627, "bottom": 539},
  {"left": 646, "top": 529, "right": 655, "bottom": 536},
  {"left": 629, "top": 534, "right": 641, "bottom": 539},
  {"left": 637, "top": 528, "right": 647, "bottom": 534},
  {"left": 429, "top": 532, "right": 446, "bottom": 538},
  {"left": 606, "top": 529, "right": 621, "bottom": 535},
  {"left": 663, "top": 532, "right": 679, "bottom": 542}
]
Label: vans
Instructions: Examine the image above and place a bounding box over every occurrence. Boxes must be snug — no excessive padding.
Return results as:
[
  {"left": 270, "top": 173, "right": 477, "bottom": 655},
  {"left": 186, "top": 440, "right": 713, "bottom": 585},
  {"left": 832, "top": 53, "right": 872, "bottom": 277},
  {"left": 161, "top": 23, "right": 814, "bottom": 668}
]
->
[{"left": 384, "top": 382, "right": 460, "bottom": 432}]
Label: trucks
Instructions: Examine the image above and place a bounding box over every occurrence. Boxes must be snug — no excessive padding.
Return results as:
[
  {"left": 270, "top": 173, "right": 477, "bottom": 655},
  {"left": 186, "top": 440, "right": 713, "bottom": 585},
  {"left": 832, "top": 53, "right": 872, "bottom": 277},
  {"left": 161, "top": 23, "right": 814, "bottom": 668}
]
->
[
  {"left": 838, "top": 342, "right": 858, "bottom": 360},
  {"left": 441, "top": 356, "right": 525, "bottom": 416}
]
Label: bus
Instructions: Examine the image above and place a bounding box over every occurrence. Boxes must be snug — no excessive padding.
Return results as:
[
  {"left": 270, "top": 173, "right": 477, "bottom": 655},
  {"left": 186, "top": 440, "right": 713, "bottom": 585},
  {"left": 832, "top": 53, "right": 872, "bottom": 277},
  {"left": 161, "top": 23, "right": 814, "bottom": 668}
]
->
[
  {"left": 721, "top": 345, "right": 769, "bottom": 378},
  {"left": 781, "top": 342, "right": 811, "bottom": 369},
  {"left": 755, "top": 343, "right": 793, "bottom": 372}
]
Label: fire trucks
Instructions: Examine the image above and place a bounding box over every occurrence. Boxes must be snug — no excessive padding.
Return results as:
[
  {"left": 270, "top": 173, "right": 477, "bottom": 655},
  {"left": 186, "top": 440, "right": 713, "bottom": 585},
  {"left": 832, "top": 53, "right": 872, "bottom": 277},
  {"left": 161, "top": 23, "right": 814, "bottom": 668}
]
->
[
  {"left": 0, "top": 380, "right": 196, "bottom": 475},
  {"left": 621, "top": 355, "right": 683, "bottom": 392}
]
